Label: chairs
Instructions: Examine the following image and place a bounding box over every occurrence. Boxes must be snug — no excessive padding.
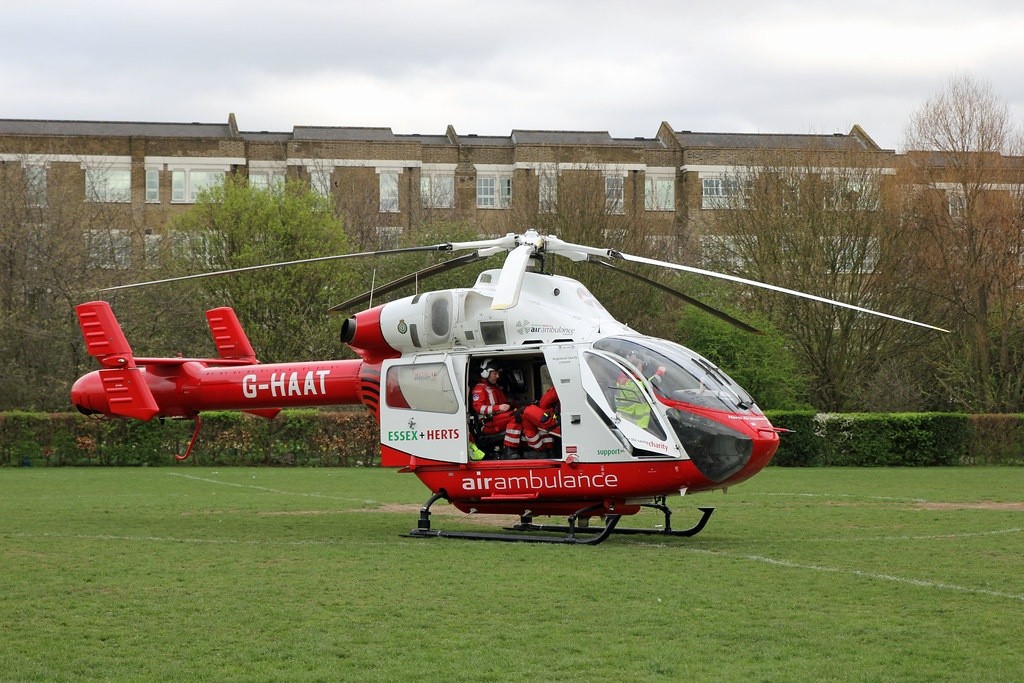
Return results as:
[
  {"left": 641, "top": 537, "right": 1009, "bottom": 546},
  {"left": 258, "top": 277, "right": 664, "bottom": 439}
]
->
[{"left": 469, "top": 401, "right": 505, "bottom": 454}]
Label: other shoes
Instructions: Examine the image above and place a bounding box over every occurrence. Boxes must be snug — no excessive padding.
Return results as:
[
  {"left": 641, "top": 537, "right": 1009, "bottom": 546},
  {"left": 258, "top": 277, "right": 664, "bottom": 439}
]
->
[
  {"left": 501, "top": 445, "right": 520, "bottom": 459},
  {"left": 523, "top": 449, "right": 549, "bottom": 459}
]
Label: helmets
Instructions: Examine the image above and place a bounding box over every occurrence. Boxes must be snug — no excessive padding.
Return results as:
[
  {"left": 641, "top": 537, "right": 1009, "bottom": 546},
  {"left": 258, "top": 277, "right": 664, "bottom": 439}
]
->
[{"left": 479, "top": 358, "right": 503, "bottom": 379}]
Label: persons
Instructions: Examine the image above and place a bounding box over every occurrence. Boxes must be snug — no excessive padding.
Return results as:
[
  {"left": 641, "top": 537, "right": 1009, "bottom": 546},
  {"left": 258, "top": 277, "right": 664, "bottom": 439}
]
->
[
  {"left": 522, "top": 385, "right": 561, "bottom": 459},
  {"left": 472, "top": 357, "right": 522, "bottom": 460},
  {"left": 614, "top": 350, "right": 666, "bottom": 429}
]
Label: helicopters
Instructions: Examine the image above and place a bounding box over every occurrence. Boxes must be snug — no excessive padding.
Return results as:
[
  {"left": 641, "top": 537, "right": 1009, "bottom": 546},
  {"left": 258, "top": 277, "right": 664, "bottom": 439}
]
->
[{"left": 66, "top": 228, "right": 950, "bottom": 551}]
